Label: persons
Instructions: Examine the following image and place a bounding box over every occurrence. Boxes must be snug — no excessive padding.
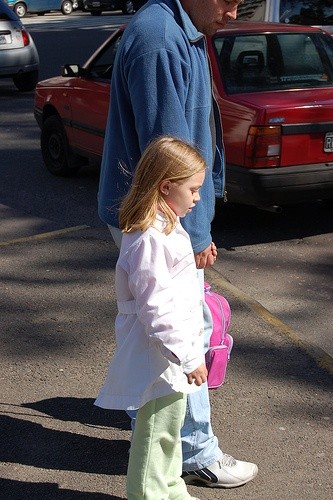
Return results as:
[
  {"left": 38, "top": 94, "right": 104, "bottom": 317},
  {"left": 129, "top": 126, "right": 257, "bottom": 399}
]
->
[
  {"left": 98, "top": 0, "right": 258, "bottom": 488},
  {"left": 94, "top": 135, "right": 217, "bottom": 500}
]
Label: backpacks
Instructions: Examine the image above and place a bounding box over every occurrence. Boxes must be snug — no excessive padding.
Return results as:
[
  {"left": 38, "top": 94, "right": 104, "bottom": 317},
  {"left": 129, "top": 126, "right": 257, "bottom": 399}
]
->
[{"left": 197, "top": 283, "right": 233, "bottom": 388}]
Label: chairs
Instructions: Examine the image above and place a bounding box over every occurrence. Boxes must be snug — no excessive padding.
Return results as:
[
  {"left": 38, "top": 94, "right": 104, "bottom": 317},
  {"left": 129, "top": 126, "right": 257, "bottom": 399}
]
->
[{"left": 230, "top": 49, "right": 267, "bottom": 87}]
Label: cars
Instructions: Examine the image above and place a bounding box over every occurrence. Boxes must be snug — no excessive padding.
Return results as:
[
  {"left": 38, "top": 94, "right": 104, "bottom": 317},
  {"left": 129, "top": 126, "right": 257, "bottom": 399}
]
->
[
  {"left": 0, "top": 0, "right": 147, "bottom": 18},
  {"left": 214, "top": 0, "right": 333, "bottom": 75},
  {"left": 0, "top": 0, "right": 41, "bottom": 92},
  {"left": 32, "top": 20, "right": 333, "bottom": 225}
]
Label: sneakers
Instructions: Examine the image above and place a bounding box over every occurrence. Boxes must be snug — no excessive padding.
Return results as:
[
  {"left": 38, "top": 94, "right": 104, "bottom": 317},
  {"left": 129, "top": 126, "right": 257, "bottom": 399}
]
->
[{"left": 180, "top": 454, "right": 258, "bottom": 488}]
